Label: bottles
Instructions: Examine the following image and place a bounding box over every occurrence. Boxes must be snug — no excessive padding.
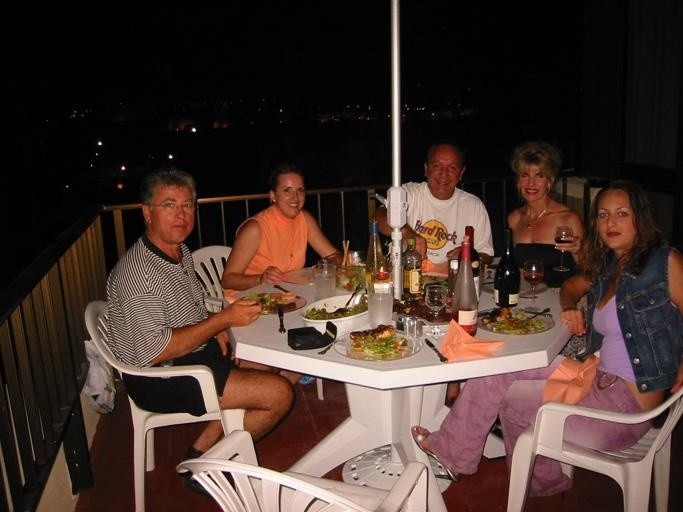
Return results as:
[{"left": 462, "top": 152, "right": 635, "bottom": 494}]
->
[
  {"left": 370, "top": 267, "right": 394, "bottom": 298},
  {"left": 452, "top": 225, "right": 479, "bottom": 336},
  {"left": 402, "top": 238, "right": 422, "bottom": 299},
  {"left": 365, "top": 221, "right": 386, "bottom": 274},
  {"left": 493, "top": 228, "right": 520, "bottom": 307}
]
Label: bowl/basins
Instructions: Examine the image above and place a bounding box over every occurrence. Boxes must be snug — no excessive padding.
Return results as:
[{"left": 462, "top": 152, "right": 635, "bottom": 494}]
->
[
  {"left": 324, "top": 251, "right": 365, "bottom": 290},
  {"left": 299, "top": 294, "right": 368, "bottom": 336}
]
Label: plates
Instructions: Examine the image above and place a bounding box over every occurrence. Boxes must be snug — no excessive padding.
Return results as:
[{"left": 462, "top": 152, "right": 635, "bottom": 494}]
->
[
  {"left": 478, "top": 307, "right": 554, "bottom": 336},
  {"left": 241, "top": 293, "right": 306, "bottom": 315},
  {"left": 335, "top": 326, "right": 423, "bottom": 361},
  {"left": 393, "top": 300, "right": 449, "bottom": 327},
  {"left": 282, "top": 270, "right": 316, "bottom": 285}
]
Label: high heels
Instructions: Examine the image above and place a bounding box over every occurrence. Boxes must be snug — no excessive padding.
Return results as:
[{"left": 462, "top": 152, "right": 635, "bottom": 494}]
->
[{"left": 412, "top": 426, "right": 461, "bottom": 482}]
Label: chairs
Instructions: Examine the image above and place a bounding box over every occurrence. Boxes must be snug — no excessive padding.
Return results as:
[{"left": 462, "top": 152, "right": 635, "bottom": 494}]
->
[
  {"left": 175, "top": 430, "right": 428, "bottom": 512},
  {"left": 505, "top": 388, "right": 683, "bottom": 512},
  {"left": 191, "top": 245, "right": 232, "bottom": 312},
  {"left": 83, "top": 301, "right": 246, "bottom": 512}
]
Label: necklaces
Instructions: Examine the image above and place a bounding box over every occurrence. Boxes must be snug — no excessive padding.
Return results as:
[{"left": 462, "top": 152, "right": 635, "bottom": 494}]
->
[{"left": 527, "top": 209, "right": 545, "bottom": 228}]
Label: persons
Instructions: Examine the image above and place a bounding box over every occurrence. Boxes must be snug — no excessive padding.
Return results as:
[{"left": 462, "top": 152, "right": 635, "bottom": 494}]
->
[
  {"left": 411, "top": 180, "right": 683, "bottom": 500},
  {"left": 221, "top": 168, "right": 345, "bottom": 388},
  {"left": 508, "top": 142, "right": 589, "bottom": 287},
  {"left": 106, "top": 169, "right": 295, "bottom": 497},
  {"left": 373, "top": 143, "right": 495, "bottom": 274}
]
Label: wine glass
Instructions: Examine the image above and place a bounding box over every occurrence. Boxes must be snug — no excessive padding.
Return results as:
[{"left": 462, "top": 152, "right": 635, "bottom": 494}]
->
[
  {"left": 523, "top": 259, "right": 545, "bottom": 312},
  {"left": 553, "top": 226, "right": 573, "bottom": 273},
  {"left": 424, "top": 285, "right": 448, "bottom": 336}
]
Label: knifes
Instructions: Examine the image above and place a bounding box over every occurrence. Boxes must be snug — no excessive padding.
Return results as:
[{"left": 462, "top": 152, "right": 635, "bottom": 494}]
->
[
  {"left": 278, "top": 304, "right": 286, "bottom": 334},
  {"left": 424, "top": 338, "right": 448, "bottom": 362}
]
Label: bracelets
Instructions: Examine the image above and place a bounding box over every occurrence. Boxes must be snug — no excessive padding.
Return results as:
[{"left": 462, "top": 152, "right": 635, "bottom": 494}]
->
[{"left": 260, "top": 272, "right": 265, "bottom": 284}]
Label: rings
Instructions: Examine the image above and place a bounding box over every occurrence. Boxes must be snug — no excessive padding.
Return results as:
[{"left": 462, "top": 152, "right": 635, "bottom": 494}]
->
[{"left": 568, "top": 320, "right": 572, "bottom": 321}]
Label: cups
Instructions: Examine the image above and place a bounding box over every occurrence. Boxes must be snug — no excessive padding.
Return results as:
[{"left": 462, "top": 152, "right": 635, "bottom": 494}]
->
[
  {"left": 313, "top": 260, "right": 338, "bottom": 299},
  {"left": 368, "top": 281, "right": 393, "bottom": 321}
]
